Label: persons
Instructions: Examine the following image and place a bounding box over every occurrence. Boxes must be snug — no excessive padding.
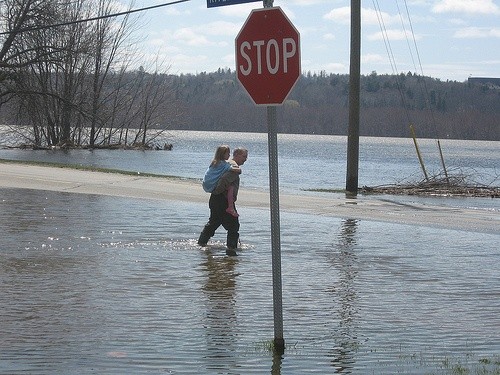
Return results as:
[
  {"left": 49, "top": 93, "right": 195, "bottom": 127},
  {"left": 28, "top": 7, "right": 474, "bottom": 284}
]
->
[
  {"left": 198, "top": 147, "right": 248, "bottom": 256},
  {"left": 203, "top": 144, "right": 241, "bottom": 218}
]
